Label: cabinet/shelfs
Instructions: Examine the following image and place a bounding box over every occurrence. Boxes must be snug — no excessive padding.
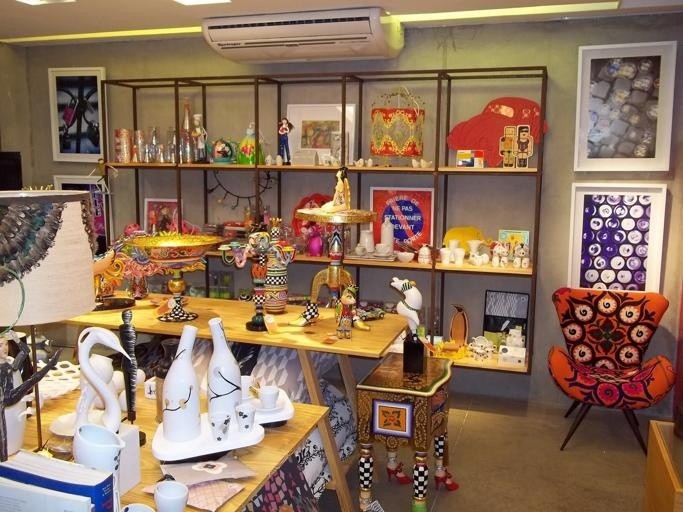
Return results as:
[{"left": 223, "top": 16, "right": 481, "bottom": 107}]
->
[
  {"left": 356, "top": 351, "right": 453, "bottom": 505},
  {"left": 100, "top": 65, "right": 546, "bottom": 377}
]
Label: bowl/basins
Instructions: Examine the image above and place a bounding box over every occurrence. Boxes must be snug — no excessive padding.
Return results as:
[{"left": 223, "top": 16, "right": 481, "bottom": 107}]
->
[{"left": 398, "top": 252, "right": 414, "bottom": 263}]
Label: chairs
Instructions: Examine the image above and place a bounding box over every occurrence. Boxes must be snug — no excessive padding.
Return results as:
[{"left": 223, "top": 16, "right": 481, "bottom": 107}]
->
[{"left": 549, "top": 286, "right": 676, "bottom": 456}]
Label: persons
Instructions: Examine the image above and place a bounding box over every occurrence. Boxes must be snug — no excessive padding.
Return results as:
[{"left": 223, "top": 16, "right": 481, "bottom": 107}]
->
[
  {"left": 0, "top": 328, "right": 63, "bottom": 462},
  {"left": 158, "top": 205, "right": 172, "bottom": 232},
  {"left": 320, "top": 167, "right": 352, "bottom": 214},
  {"left": 277, "top": 117, "right": 294, "bottom": 165},
  {"left": 190, "top": 114, "right": 208, "bottom": 161},
  {"left": 379, "top": 430, "right": 459, "bottom": 493},
  {"left": 235, "top": 121, "right": 267, "bottom": 165}
]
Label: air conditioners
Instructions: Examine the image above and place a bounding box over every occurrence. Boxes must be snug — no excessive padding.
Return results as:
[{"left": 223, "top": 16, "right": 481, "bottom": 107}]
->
[{"left": 203, "top": 8, "right": 403, "bottom": 67}]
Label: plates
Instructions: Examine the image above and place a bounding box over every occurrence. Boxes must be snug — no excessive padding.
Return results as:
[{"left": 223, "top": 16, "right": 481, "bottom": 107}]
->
[{"left": 49, "top": 408, "right": 105, "bottom": 437}]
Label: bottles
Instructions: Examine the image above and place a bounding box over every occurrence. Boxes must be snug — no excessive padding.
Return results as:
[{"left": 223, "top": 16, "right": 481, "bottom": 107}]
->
[
  {"left": 160, "top": 316, "right": 243, "bottom": 445},
  {"left": 380, "top": 217, "right": 395, "bottom": 255},
  {"left": 182, "top": 97, "right": 205, "bottom": 163}
]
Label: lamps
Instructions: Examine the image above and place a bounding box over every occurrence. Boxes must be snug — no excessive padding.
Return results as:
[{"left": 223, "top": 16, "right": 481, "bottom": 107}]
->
[{"left": 0, "top": 188, "right": 96, "bottom": 463}]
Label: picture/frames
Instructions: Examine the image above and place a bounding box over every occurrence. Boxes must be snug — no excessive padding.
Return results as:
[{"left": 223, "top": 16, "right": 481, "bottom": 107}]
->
[
  {"left": 45, "top": 66, "right": 111, "bottom": 260},
  {"left": 568, "top": 41, "right": 675, "bottom": 302}
]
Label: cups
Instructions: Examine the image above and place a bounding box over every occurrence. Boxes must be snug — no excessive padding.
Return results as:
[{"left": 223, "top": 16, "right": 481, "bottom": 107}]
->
[
  {"left": 119, "top": 502, "right": 157, "bottom": 512},
  {"left": 440, "top": 238, "right": 530, "bottom": 269},
  {"left": 239, "top": 375, "right": 253, "bottom": 398},
  {"left": 234, "top": 403, "right": 256, "bottom": 436},
  {"left": 71, "top": 424, "right": 127, "bottom": 512},
  {"left": 354, "top": 222, "right": 391, "bottom": 258},
  {"left": 144, "top": 124, "right": 199, "bottom": 164},
  {"left": 154, "top": 481, "right": 190, "bottom": 512},
  {"left": 3, "top": 331, "right": 34, "bottom": 456},
  {"left": 259, "top": 386, "right": 279, "bottom": 408}
]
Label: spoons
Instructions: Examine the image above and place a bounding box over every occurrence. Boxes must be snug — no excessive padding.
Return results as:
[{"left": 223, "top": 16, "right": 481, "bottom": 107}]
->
[{"left": 48, "top": 443, "right": 73, "bottom": 457}]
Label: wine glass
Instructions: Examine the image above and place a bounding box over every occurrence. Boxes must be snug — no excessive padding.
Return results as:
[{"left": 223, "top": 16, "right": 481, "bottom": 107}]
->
[{"left": 124, "top": 234, "right": 228, "bottom": 322}]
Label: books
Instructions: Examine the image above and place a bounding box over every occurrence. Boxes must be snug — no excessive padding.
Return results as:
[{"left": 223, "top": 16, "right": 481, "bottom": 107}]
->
[{"left": 0, "top": 449, "right": 114, "bottom": 511}]
